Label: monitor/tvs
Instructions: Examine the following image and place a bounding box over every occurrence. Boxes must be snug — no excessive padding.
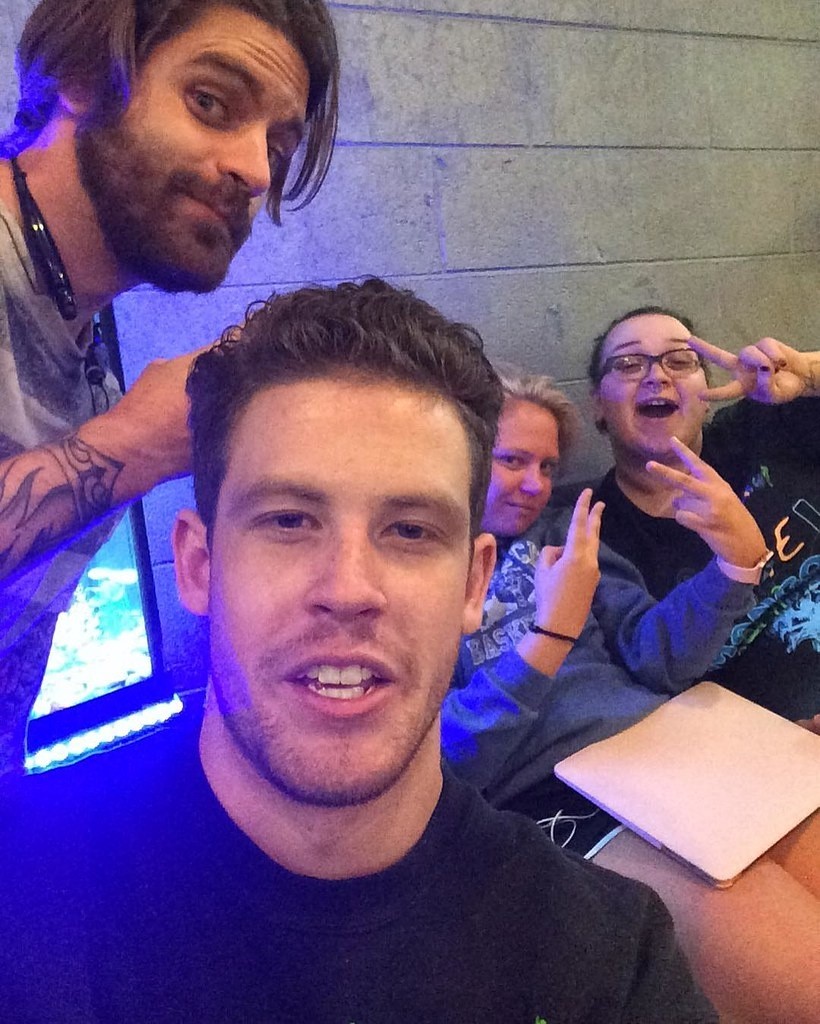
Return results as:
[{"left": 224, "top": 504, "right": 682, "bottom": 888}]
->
[{"left": 28, "top": 506, "right": 154, "bottom": 723}]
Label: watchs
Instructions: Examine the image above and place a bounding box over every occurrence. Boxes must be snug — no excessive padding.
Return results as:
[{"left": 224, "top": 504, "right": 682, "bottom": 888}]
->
[{"left": 717, "top": 548, "right": 777, "bottom": 587}]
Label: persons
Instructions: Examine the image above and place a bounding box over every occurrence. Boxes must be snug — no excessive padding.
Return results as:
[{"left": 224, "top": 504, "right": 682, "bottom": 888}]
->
[
  {"left": 0, "top": 0, "right": 340, "bottom": 773},
  {"left": 0, "top": 274, "right": 717, "bottom": 1024},
  {"left": 439, "top": 362, "right": 820, "bottom": 1024},
  {"left": 587, "top": 304, "right": 820, "bottom": 736}
]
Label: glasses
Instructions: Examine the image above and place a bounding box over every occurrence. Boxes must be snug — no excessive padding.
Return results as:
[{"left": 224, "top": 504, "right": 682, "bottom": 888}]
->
[{"left": 599, "top": 348, "right": 703, "bottom": 380}]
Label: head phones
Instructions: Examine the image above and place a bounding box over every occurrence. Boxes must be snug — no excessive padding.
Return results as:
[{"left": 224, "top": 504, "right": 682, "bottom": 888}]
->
[{"left": 11, "top": 156, "right": 110, "bottom": 384}]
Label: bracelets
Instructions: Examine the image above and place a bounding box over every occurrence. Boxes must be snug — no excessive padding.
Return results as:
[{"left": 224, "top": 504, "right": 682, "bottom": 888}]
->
[{"left": 528, "top": 620, "right": 579, "bottom": 643}]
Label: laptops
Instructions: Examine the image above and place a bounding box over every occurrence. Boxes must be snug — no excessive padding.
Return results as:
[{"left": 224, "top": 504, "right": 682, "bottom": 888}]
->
[{"left": 554, "top": 681, "right": 820, "bottom": 889}]
